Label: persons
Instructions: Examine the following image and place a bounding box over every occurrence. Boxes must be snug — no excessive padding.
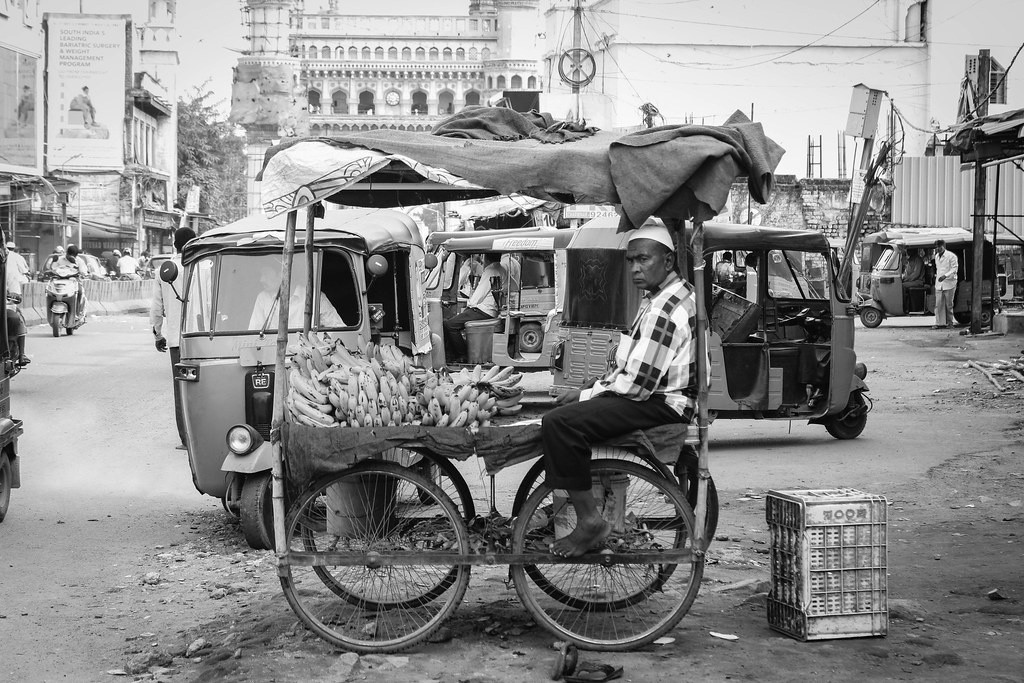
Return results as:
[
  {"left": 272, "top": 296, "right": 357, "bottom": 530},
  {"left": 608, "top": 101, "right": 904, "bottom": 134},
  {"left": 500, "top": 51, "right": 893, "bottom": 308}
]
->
[
  {"left": 150, "top": 227, "right": 213, "bottom": 450},
  {"left": 445, "top": 249, "right": 520, "bottom": 360},
  {"left": 541, "top": 217, "right": 711, "bottom": 559},
  {"left": 246, "top": 257, "right": 348, "bottom": 330},
  {"left": 4, "top": 241, "right": 150, "bottom": 319},
  {"left": 902, "top": 245, "right": 925, "bottom": 313},
  {"left": 931, "top": 240, "right": 959, "bottom": 329},
  {"left": 714, "top": 252, "right": 734, "bottom": 282}
]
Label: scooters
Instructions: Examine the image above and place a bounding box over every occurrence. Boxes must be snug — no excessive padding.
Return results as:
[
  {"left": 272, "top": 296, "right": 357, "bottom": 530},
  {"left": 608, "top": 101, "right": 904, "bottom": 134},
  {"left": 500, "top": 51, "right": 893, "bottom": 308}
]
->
[
  {"left": 7, "top": 292, "right": 27, "bottom": 377},
  {"left": 44, "top": 256, "right": 88, "bottom": 337},
  {"left": 105, "top": 268, "right": 145, "bottom": 281}
]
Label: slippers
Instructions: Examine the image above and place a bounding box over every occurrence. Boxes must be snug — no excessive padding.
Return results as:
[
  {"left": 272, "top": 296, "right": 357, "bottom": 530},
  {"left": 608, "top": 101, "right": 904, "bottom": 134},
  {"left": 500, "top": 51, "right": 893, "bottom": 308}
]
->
[
  {"left": 574, "top": 660, "right": 624, "bottom": 683},
  {"left": 552, "top": 640, "right": 578, "bottom": 680}
]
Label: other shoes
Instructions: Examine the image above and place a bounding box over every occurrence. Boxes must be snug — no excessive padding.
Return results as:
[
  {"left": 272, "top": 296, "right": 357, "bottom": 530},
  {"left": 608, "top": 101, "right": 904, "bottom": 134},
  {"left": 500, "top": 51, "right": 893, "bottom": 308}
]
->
[{"left": 932, "top": 325, "right": 954, "bottom": 329}]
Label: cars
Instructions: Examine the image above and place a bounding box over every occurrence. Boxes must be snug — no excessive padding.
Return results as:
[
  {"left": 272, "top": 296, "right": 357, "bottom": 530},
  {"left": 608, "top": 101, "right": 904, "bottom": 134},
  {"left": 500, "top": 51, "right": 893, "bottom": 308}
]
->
[{"left": 144, "top": 253, "right": 175, "bottom": 280}]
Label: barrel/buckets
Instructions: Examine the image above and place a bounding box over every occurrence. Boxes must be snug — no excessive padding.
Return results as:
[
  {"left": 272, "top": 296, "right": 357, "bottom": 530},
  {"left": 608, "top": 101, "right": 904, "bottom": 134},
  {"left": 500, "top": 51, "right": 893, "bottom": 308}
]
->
[
  {"left": 320, "top": 460, "right": 399, "bottom": 539},
  {"left": 551, "top": 472, "right": 630, "bottom": 541}
]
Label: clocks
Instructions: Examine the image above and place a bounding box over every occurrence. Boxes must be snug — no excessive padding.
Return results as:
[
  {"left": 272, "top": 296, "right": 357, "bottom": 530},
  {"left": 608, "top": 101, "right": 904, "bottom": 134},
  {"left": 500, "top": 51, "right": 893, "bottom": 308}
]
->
[{"left": 386, "top": 92, "right": 400, "bottom": 106}]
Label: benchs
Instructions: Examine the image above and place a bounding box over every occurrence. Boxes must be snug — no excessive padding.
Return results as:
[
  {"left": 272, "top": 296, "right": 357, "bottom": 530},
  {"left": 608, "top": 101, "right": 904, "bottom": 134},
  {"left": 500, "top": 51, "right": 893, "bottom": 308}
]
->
[
  {"left": 909, "top": 286, "right": 927, "bottom": 312},
  {"left": 463, "top": 318, "right": 503, "bottom": 364},
  {"left": 769, "top": 346, "right": 801, "bottom": 405}
]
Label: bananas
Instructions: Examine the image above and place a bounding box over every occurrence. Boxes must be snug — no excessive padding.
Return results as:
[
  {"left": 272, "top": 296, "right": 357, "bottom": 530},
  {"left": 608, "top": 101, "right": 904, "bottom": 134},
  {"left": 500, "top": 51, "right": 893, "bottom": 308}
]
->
[{"left": 286, "top": 332, "right": 523, "bottom": 428}]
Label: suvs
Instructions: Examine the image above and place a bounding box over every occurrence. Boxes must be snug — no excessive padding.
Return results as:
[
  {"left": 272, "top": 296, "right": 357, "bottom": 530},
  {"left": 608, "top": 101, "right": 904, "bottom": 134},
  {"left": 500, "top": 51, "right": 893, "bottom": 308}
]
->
[{"left": 38, "top": 252, "right": 116, "bottom": 282}]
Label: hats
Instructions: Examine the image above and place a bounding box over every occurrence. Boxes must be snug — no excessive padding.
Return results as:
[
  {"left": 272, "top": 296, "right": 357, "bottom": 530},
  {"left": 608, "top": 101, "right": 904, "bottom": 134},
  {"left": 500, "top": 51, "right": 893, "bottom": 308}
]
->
[
  {"left": 6, "top": 241, "right": 15, "bottom": 248},
  {"left": 627, "top": 223, "right": 674, "bottom": 252}
]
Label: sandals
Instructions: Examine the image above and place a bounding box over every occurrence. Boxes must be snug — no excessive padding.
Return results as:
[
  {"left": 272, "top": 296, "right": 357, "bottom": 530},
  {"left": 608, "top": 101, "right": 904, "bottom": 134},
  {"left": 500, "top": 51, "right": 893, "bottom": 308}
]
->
[{"left": 19, "top": 354, "right": 31, "bottom": 363}]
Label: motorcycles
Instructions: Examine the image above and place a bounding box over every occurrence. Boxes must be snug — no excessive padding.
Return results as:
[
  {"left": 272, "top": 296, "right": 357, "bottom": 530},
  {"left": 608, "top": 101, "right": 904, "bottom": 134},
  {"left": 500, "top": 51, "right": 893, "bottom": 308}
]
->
[
  {"left": 853, "top": 226, "right": 1007, "bottom": 326},
  {"left": 157, "top": 208, "right": 442, "bottom": 551},
  {"left": 427, "top": 217, "right": 874, "bottom": 441}
]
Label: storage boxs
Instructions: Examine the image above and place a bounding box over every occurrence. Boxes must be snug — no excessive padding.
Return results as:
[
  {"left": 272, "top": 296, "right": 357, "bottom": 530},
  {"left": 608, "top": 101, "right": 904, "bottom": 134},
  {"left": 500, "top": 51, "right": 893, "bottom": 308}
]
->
[{"left": 764, "top": 487, "right": 890, "bottom": 642}]
